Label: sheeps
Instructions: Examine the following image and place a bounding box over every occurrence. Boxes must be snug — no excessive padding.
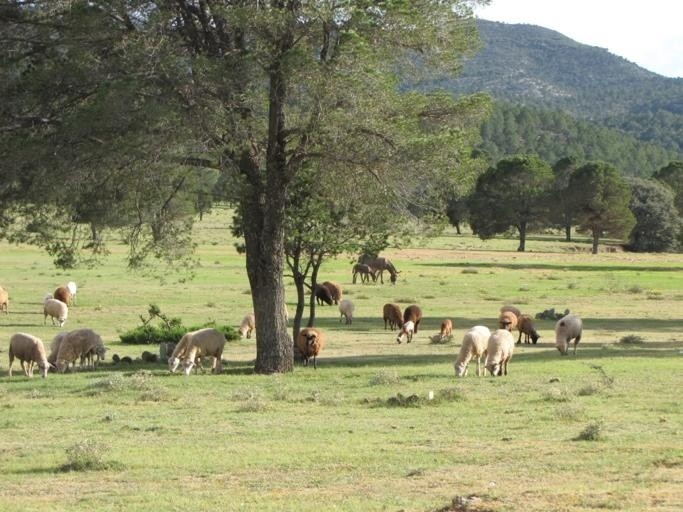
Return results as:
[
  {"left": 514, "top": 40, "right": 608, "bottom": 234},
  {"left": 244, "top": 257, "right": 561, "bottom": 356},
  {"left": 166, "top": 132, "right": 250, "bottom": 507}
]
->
[
  {"left": 499, "top": 304, "right": 540, "bottom": 346},
  {"left": 43, "top": 282, "right": 78, "bottom": 328},
  {"left": 555, "top": 314, "right": 584, "bottom": 355},
  {"left": 484, "top": 328, "right": 515, "bottom": 376},
  {"left": 238, "top": 312, "right": 255, "bottom": 339},
  {"left": 454, "top": 325, "right": 490, "bottom": 378},
  {"left": 314, "top": 280, "right": 356, "bottom": 327},
  {"left": 439, "top": 319, "right": 452, "bottom": 338},
  {"left": 382, "top": 304, "right": 422, "bottom": 345},
  {"left": 168, "top": 331, "right": 206, "bottom": 375},
  {"left": 296, "top": 328, "right": 326, "bottom": 370},
  {"left": 7, "top": 329, "right": 109, "bottom": 380},
  {"left": 179, "top": 328, "right": 225, "bottom": 376},
  {"left": 0, "top": 286, "right": 10, "bottom": 314}
]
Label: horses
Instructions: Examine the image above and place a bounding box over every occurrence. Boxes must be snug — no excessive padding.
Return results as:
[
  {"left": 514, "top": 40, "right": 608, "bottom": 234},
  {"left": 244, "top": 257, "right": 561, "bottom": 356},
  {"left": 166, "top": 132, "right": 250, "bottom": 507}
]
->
[
  {"left": 357, "top": 255, "right": 400, "bottom": 285},
  {"left": 352, "top": 263, "right": 377, "bottom": 285}
]
